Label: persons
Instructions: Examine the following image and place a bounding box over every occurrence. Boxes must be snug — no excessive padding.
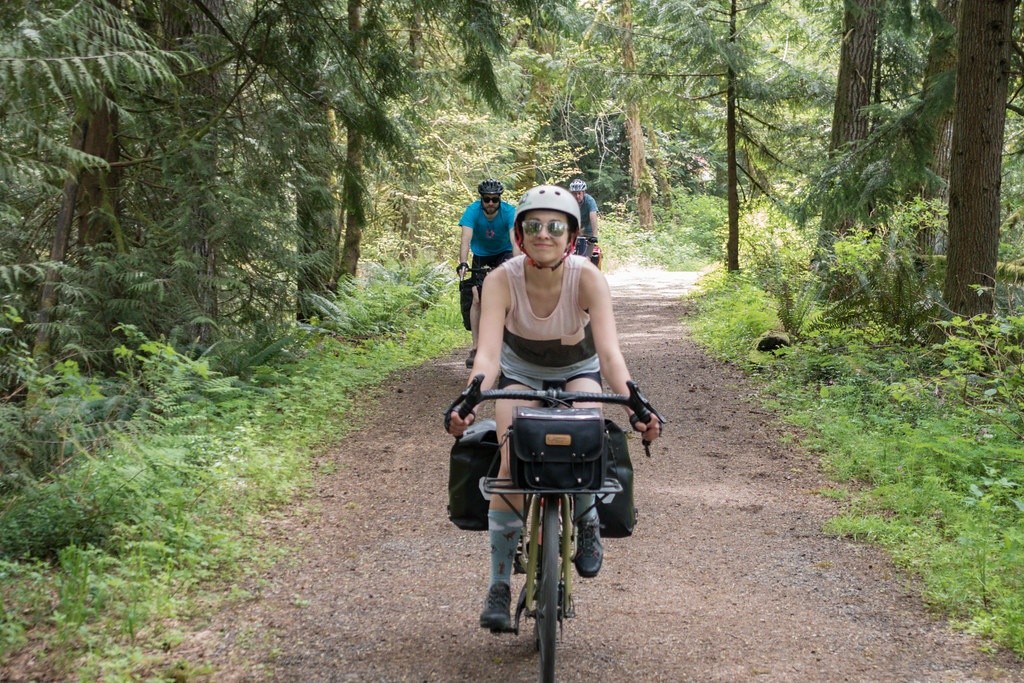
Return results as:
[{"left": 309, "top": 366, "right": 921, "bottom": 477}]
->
[
  {"left": 458, "top": 179, "right": 522, "bottom": 368},
  {"left": 569, "top": 179, "right": 599, "bottom": 260},
  {"left": 450, "top": 185, "right": 659, "bottom": 628}
]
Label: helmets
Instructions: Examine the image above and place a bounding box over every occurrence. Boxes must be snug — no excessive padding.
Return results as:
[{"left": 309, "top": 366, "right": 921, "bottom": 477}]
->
[
  {"left": 477, "top": 179, "right": 505, "bottom": 195},
  {"left": 513, "top": 185, "right": 581, "bottom": 240},
  {"left": 569, "top": 179, "right": 588, "bottom": 192}
]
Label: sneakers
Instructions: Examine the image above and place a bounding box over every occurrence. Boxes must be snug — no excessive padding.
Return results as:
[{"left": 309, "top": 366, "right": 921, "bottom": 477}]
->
[
  {"left": 479, "top": 583, "right": 512, "bottom": 630},
  {"left": 574, "top": 516, "right": 603, "bottom": 578}
]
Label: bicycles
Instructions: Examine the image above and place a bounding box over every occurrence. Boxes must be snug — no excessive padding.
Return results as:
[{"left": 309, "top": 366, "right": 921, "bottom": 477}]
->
[
  {"left": 459, "top": 262, "right": 513, "bottom": 301},
  {"left": 572, "top": 235, "right": 602, "bottom": 267},
  {"left": 443, "top": 373, "right": 668, "bottom": 683}
]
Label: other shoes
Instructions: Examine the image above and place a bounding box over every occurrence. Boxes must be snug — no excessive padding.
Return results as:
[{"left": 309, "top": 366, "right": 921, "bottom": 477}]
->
[{"left": 465, "top": 349, "right": 477, "bottom": 369}]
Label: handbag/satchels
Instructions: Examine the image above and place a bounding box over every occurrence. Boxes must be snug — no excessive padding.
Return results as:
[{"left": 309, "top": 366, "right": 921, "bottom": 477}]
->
[
  {"left": 508, "top": 401, "right": 606, "bottom": 494},
  {"left": 459, "top": 277, "right": 472, "bottom": 331},
  {"left": 596, "top": 418, "right": 639, "bottom": 538},
  {"left": 446, "top": 419, "right": 501, "bottom": 531}
]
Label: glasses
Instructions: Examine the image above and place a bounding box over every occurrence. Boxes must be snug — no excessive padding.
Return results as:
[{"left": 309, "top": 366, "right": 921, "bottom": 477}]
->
[
  {"left": 481, "top": 196, "right": 499, "bottom": 204},
  {"left": 521, "top": 220, "right": 566, "bottom": 238}
]
